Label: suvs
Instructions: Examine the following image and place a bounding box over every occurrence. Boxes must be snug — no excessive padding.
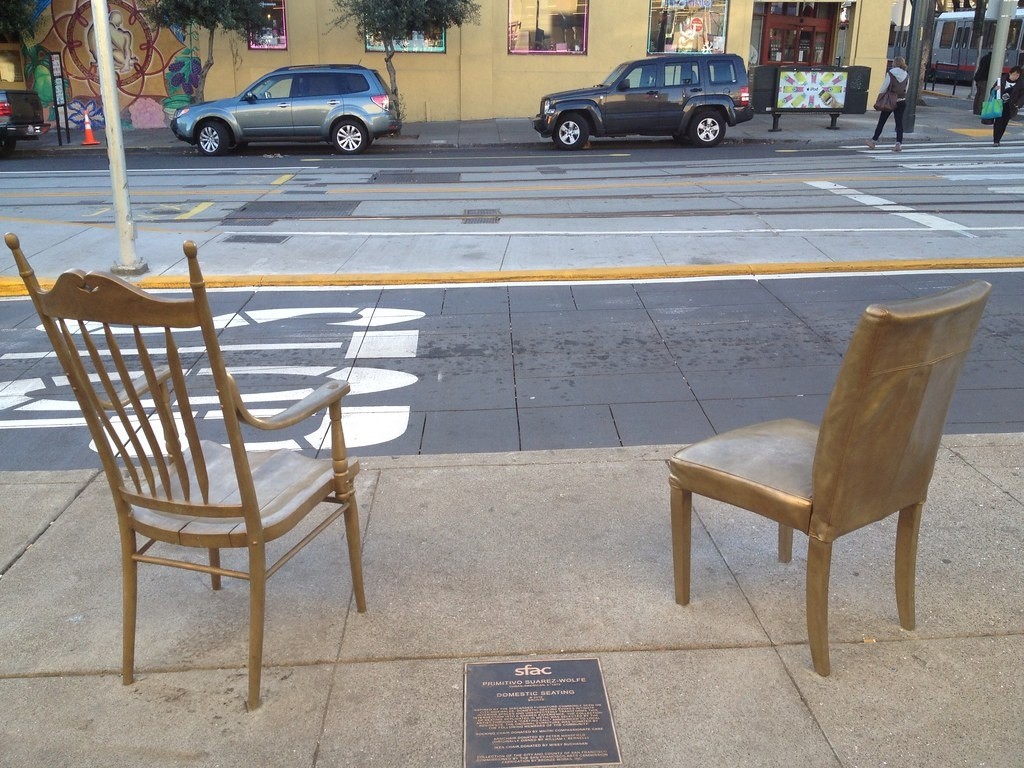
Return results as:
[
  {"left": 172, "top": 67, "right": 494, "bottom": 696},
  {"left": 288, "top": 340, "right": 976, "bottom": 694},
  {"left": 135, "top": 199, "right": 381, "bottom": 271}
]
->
[
  {"left": 170, "top": 64, "right": 402, "bottom": 156},
  {"left": 533, "top": 53, "right": 754, "bottom": 151}
]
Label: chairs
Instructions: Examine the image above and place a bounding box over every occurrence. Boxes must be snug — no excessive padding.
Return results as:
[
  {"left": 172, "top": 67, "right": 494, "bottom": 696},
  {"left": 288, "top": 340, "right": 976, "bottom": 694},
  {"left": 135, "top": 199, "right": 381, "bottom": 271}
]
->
[
  {"left": 4, "top": 234, "right": 367, "bottom": 711},
  {"left": 663, "top": 280, "right": 990, "bottom": 678}
]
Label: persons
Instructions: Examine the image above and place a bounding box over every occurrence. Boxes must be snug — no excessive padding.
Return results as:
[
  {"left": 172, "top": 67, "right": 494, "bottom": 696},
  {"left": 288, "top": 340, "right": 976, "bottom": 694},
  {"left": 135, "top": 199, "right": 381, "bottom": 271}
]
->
[
  {"left": 973, "top": 52, "right": 992, "bottom": 115},
  {"left": 559, "top": 10, "right": 577, "bottom": 51},
  {"left": 990, "top": 66, "right": 1024, "bottom": 146},
  {"left": 865, "top": 56, "right": 910, "bottom": 151},
  {"left": 679, "top": 16, "right": 696, "bottom": 52}
]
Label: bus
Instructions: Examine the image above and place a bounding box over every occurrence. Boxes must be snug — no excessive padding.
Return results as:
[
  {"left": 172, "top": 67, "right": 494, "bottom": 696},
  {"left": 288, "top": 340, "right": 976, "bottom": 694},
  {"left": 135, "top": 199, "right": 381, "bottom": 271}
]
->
[{"left": 836, "top": 10, "right": 1024, "bottom": 86}]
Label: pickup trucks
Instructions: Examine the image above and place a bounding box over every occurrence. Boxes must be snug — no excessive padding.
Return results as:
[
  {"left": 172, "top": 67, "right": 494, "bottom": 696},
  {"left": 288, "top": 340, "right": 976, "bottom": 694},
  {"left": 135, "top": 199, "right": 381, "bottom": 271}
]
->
[{"left": 0, "top": 89, "right": 51, "bottom": 156}]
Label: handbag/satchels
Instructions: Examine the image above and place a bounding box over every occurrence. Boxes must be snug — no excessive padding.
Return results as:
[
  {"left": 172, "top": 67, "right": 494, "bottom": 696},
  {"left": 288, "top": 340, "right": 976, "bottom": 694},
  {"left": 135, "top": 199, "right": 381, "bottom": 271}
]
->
[
  {"left": 874, "top": 72, "right": 897, "bottom": 112},
  {"left": 980, "top": 87, "right": 1003, "bottom": 125}
]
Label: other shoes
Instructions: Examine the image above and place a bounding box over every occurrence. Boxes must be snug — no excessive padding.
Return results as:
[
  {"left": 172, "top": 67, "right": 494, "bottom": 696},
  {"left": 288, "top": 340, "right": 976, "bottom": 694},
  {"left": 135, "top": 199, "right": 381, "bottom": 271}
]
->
[
  {"left": 894, "top": 141, "right": 902, "bottom": 151},
  {"left": 993, "top": 140, "right": 1000, "bottom": 147},
  {"left": 864, "top": 140, "right": 876, "bottom": 149}
]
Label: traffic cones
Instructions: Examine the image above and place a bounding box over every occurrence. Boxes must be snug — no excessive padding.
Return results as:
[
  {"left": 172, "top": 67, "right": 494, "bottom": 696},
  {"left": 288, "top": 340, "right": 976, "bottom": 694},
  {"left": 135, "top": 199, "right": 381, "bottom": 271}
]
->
[{"left": 81, "top": 110, "right": 101, "bottom": 145}]
